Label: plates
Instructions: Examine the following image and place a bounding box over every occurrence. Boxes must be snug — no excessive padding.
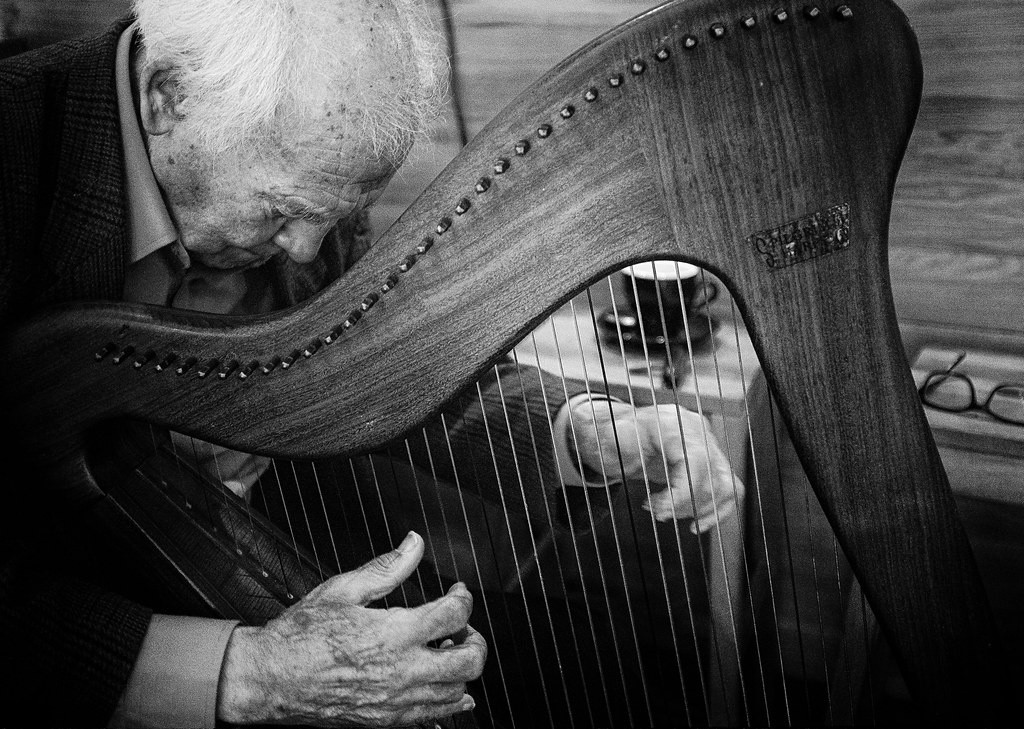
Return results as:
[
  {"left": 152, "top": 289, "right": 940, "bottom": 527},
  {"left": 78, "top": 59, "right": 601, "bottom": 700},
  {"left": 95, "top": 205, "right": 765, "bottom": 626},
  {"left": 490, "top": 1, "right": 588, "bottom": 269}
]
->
[{"left": 598, "top": 303, "right": 719, "bottom": 355}]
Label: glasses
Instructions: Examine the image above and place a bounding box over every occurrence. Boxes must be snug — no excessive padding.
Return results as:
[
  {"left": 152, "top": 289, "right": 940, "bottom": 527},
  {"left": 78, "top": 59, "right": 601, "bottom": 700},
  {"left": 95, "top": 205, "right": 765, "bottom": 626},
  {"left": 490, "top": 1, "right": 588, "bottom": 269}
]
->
[{"left": 917, "top": 351, "right": 1024, "bottom": 427}]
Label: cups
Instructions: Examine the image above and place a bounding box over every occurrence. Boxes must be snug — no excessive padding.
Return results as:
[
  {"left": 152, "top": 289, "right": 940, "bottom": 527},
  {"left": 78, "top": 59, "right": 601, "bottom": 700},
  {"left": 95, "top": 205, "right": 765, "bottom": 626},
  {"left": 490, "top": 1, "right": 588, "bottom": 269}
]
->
[{"left": 621, "top": 259, "right": 719, "bottom": 334}]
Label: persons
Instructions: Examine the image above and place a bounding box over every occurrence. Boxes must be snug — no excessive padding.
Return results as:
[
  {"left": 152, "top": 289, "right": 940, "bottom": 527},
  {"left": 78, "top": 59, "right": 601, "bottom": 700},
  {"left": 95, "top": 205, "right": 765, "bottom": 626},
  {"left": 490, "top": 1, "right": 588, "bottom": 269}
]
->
[{"left": 0, "top": 0, "right": 746, "bottom": 729}]
